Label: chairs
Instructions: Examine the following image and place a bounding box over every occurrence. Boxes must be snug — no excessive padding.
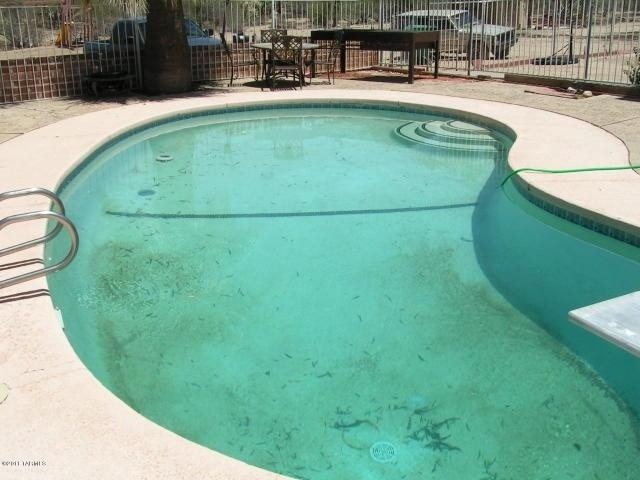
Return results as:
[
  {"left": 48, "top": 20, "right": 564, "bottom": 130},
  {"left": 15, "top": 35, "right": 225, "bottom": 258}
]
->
[{"left": 219, "top": 29, "right": 343, "bottom": 91}]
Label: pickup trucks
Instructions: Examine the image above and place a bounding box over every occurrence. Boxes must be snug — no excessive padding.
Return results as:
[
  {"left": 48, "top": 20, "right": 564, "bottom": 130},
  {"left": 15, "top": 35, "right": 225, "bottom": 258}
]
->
[{"left": 83, "top": 17, "right": 225, "bottom": 55}]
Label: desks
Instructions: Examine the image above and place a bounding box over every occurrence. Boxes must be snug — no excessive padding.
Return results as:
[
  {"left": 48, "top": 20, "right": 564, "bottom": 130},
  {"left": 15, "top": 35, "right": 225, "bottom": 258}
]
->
[{"left": 311, "top": 30, "right": 439, "bottom": 84}]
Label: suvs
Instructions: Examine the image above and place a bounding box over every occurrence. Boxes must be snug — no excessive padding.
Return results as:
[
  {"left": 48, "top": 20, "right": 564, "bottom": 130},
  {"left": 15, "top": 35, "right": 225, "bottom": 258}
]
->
[{"left": 390, "top": 9, "right": 517, "bottom": 61}]
